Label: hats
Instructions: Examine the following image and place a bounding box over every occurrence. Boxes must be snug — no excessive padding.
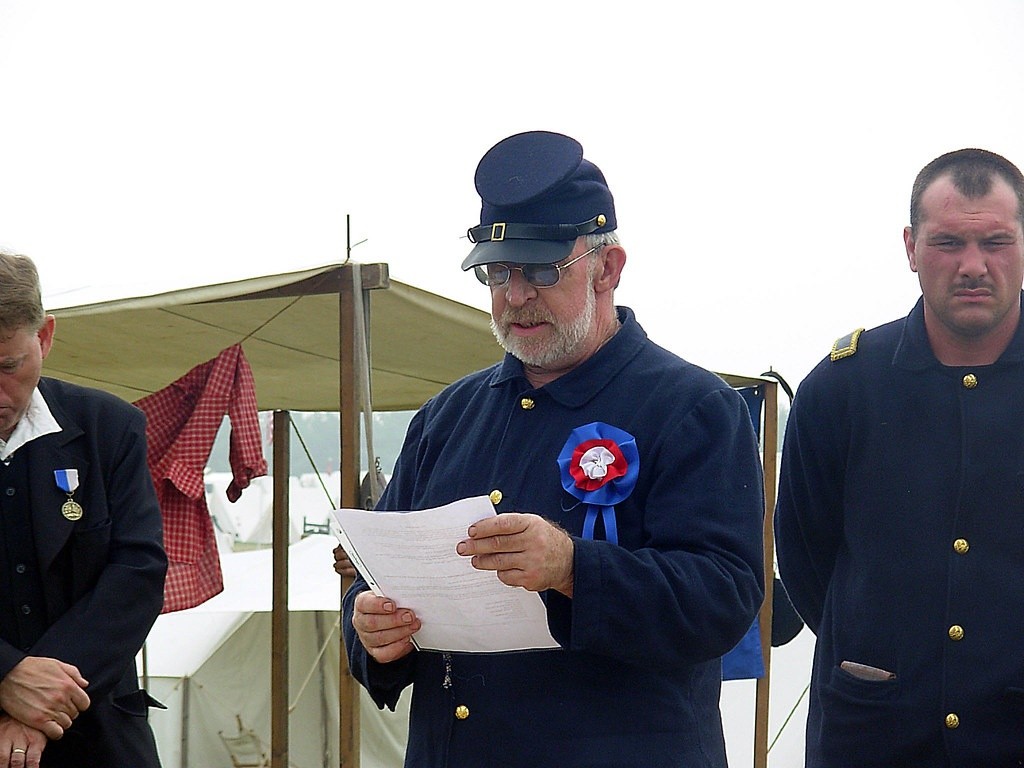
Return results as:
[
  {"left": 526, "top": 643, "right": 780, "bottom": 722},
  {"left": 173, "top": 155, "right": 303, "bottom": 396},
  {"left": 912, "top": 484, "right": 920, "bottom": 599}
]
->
[{"left": 461, "top": 130, "right": 617, "bottom": 271}]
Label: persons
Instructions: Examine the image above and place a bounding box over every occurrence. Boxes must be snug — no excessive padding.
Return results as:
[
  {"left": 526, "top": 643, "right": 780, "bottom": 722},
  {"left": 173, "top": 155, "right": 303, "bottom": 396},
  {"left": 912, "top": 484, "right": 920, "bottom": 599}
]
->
[
  {"left": 332, "top": 542, "right": 359, "bottom": 581},
  {"left": 773, "top": 147, "right": 1024, "bottom": 768},
  {"left": 0, "top": 248, "right": 168, "bottom": 768},
  {"left": 344, "top": 129, "right": 764, "bottom": 768}
]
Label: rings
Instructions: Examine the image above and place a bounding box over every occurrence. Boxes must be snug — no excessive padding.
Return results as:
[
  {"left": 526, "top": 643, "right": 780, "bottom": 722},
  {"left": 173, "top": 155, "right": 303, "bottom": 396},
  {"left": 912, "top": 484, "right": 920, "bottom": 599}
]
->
[{"left": 11, "top": 748, "right": 26, "bottom": 754}]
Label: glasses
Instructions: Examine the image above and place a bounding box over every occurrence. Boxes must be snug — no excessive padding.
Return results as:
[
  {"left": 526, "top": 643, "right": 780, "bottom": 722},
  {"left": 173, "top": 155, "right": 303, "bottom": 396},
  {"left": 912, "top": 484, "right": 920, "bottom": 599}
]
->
[{"left": 474, "top": 240, "right": 607, "bottom": 289}]
